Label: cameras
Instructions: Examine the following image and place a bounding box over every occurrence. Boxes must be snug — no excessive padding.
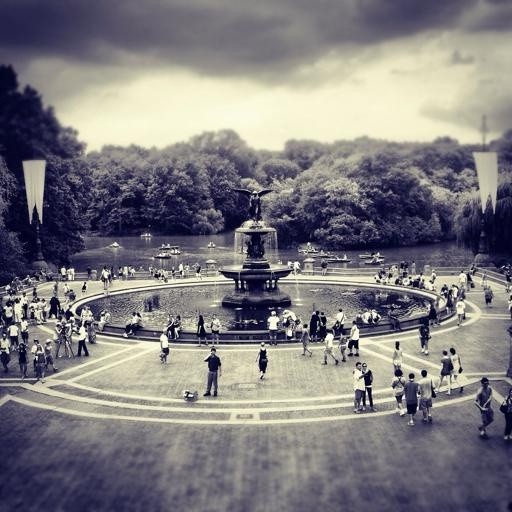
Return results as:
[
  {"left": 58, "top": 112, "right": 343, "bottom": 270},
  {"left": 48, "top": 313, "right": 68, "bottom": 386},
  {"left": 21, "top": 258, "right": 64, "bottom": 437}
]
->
[{"left": 61, "top": 325, "right": 68, "bottom": 330}]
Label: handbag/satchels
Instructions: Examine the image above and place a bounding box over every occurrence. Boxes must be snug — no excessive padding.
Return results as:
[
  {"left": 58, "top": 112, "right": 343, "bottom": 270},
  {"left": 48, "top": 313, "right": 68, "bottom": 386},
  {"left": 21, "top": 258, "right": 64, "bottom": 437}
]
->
[
  {"left": 432, "top": 390, "right": 436, "bottom": 398},
  {"left": 500, "top": 404, "right": 508, "bottom": 413},
  {"left": 458, "top": 367, "right": 463, "bottom": 373},
  {"left": 261, "top": 359, "right": 267, "bottom": 363}
]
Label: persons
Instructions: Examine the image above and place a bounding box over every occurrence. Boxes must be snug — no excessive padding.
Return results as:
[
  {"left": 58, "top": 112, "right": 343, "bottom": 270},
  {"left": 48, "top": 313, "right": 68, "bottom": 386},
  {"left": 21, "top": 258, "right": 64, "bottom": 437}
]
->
[
  {"left": 1, "top": 240, "right": 512, "bottom": 425},
  {"left": 474, "top": 375, "right": 512, "bottom": 444},
  {"left": 248, "top": 191, "right": 261, "bottom": 223}
]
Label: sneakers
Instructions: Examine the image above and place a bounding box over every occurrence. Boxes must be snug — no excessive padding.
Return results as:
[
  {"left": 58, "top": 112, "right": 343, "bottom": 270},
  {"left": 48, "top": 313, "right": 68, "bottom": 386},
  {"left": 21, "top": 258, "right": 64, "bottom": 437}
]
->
[
  {"left": 354, "top": 408, "right": 368, "bottom": 413},
  {"left": 204, "top": 392, "right": 211, "bottom": 396},
  {"left": 348, "top": 353, "right": 353, "bottom": 357},
  {"left": 408, "top": 421, "right": 415, "bottom": 426},
  {"left": 371, "top": 409, "right": 377, "bottom": 412},
  {"left": 424, "top": 420, "right": 427, "bottom": 423},
  {"left": 213, "top": 392, "right": 217, "bottom": 396},
  {"left": 396, "top": 408, "right": 406, "bottom": 417},
  {"left": 54, "top": 368, "right": 58, "bottom": 372},
  {"left": 479, "top": 426, "right": 488, "bottom": 440},
  {"left": 428, "top": 416, "right": 432, "bottom": 422},
  {"left": 354, "top": 353, "right": 359, "bottom": 356},
  {"left": 504, "top": 435, "right": 512, "bottom": 440}
]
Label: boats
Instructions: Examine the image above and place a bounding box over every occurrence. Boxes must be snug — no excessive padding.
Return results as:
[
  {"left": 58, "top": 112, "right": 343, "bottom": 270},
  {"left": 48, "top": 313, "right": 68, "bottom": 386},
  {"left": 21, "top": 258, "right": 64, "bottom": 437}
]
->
[
  {"left": 296, "top": 246, "right": 389, "bottom": 267},
  {"left": 140, "top": 233, "right": 152, "bottom": 238},
  {"left": 109, "top": 242, "right": 122, "bottom": 249},
  {"left": 206, "top": 239, "right": 218, "bottom": 250},
  {"left": 153, "top": 241, "right": 182, "bottom": 261}
]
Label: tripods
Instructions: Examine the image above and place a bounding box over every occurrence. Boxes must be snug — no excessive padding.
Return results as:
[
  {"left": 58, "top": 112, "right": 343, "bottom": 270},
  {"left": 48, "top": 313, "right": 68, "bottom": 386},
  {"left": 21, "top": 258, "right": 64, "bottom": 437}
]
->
[{"left": 55, "top": 335, "right": 76, "bottom": 359}]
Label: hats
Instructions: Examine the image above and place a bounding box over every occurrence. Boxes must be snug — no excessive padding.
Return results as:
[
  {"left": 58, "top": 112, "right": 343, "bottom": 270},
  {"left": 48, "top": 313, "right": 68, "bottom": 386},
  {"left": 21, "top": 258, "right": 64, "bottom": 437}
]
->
[{"left": 46, "top": 339, "right": 53, "bottom": 344}]
